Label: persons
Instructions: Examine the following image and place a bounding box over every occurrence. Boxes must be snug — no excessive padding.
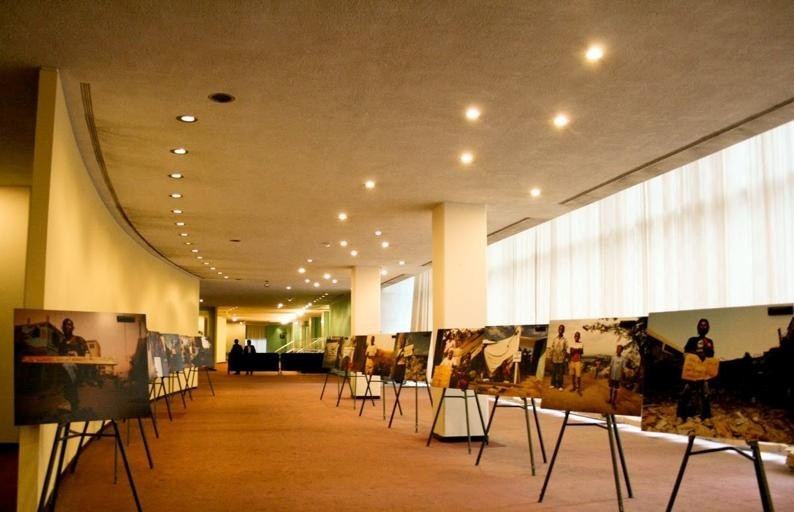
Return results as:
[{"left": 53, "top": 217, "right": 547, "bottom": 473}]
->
[
  {"left": 394, "top": 337, "right": 414, "bottom": 380},
  {"left": 673, "top": 318, "right": 714, "bottom": 429},
  {"left": 568, "top": 332, "right": 584, "bottom": 397},
  {"left": 594, "top": 355, "right": 601, "bottom": 378},
  {"left": 604, "top": 345, "right": 625, "bottom": 410},
  {"left": 231, "top": 339, "right": 243, "bottom": 375},
  {"left": 439, "top": 332, "right": 471, "bottom": 368},
  {"left": 549, "top": 325, "right": 567, "bottom": 391},
  {"left": 56, "top": 318, "right": 91, "bottom": 411},
  {"left": 364, "top": 336, "right": 377, "bottom": 375},
  {"left": 244, "top": 340, "right": 256, "bottom": 375}
]
